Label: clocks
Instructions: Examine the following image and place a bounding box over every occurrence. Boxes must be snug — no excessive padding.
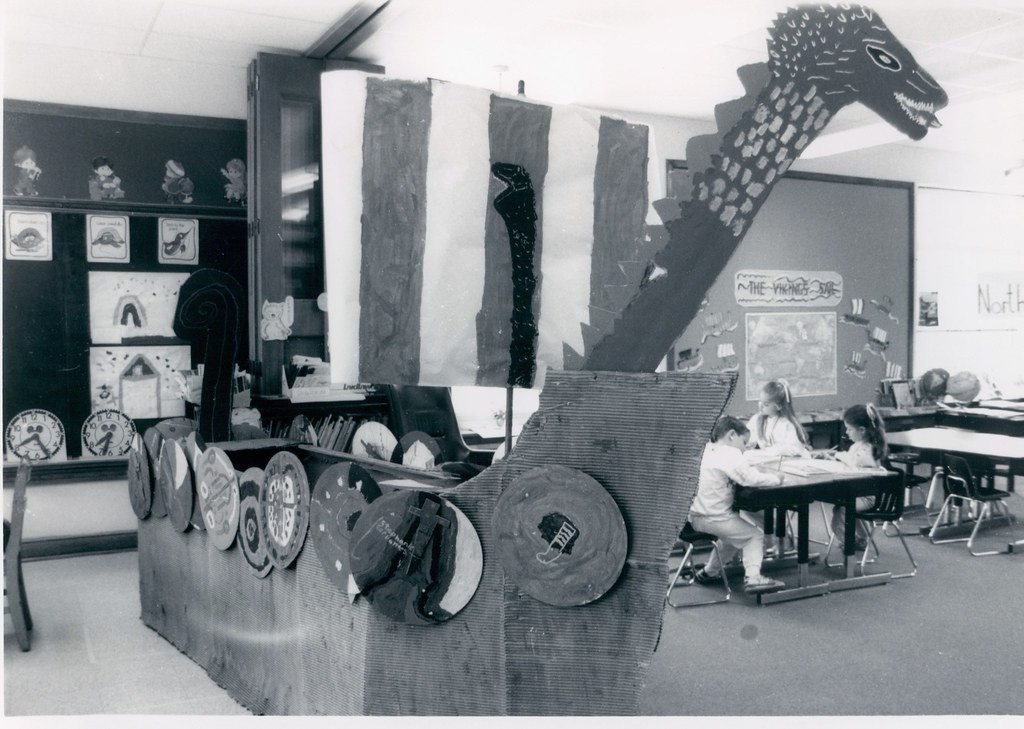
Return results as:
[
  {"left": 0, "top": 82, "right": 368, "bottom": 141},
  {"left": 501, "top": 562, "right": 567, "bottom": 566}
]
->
[
  {"left": 81, "top": 408, "right": 138, "bottom": 460},
  {"left": 5, "top": 409, "right": 68, "bottom": 464}
]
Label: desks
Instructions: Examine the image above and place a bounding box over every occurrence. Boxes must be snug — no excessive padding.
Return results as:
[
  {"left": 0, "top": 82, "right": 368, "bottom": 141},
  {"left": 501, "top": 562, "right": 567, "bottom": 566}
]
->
[
  {"left": 937, "top": 406, "right": 1024, "bottom": 439},
  {"left": 728, "top": 410, "right": 840, "bottom": 452},
  {"left": 730, "top": 457, "right": 900, "bottom": 606},
  {"left": 885, "top": 427, "right": 1024, "bottom": 553},
  {"left": 827, "top": 407, "right": 943, "bottom": 450}
]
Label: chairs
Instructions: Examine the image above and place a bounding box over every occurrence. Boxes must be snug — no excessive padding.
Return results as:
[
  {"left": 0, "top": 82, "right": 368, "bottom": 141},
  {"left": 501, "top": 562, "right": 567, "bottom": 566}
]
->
[
  {"left": 667, "top": 450, "right": 929, "bottom": 606},
  {"left": 928, "top": 454, "right": 1018, "bottom": 557},
  {"left": 842, "top": 409, "right": 1024, "bottom": 536}
]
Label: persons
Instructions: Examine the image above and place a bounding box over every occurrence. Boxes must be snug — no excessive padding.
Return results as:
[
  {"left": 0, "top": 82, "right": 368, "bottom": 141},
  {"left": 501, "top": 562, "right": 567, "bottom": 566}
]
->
[
  {"left": 689, "top": 414, "right": 787, "bottom": 594},
  {"left": 809, "top": 404, "right": 887, "bottom": 556},
  {"left": 746, "top": 379, "right": 810, "bottom": 561}
]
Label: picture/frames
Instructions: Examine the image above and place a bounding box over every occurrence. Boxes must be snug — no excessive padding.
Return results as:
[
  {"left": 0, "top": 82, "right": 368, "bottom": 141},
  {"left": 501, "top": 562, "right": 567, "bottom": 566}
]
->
[
  {"left": 85, "top": 214, "right": 131, "bottom": 263},
  {"left": 4, "top": 210, "right": 54, "bottom": 261},
  {"left": 158, "top": 218, "right": 199, "bottom": 265}
]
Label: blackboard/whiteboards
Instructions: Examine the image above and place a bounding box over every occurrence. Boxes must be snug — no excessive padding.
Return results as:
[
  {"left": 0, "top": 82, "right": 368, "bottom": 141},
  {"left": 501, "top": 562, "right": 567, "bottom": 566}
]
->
[{"left": 665, "top": 159, "right": 915, "bottom": 416}]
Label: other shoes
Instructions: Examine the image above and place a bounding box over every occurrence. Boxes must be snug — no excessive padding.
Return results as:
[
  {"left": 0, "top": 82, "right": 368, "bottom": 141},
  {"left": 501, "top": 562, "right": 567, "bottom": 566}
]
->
[
  {"left": 744, "top": 574, "right": 785, "bottom": 595},
  {"left": 696, "top": 568, "right": 724, "bottom": 581}
]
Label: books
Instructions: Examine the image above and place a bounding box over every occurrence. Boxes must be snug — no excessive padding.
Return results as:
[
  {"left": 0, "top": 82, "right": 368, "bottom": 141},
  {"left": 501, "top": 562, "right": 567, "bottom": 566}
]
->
[
  {"left": 261, "top": 413, "right": 391, "bottom": 456},
  {"left": 881, "top": 377, "right": 924, "bottom": 409}
]
